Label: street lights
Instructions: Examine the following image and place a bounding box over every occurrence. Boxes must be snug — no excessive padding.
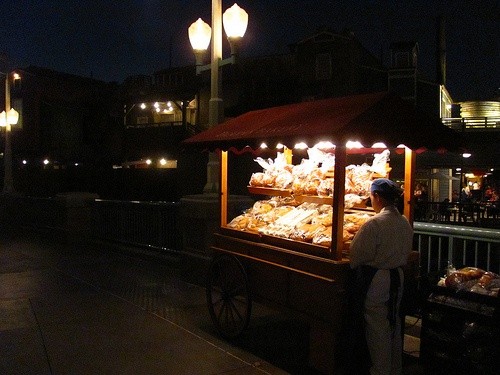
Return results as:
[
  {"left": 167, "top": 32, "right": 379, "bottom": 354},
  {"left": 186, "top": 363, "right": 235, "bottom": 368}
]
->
[
  {"left": 188, "top": 0, "right": 249, "bottom": 196},
  {"left": 1, "top": 71, "right": 22, "bottom": 196}
]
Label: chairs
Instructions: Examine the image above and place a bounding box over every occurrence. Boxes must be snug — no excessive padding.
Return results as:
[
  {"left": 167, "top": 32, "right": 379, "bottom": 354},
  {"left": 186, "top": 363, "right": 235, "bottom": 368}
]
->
[{"left": 458, "top": 209, "right": 476, "bottom": 223}]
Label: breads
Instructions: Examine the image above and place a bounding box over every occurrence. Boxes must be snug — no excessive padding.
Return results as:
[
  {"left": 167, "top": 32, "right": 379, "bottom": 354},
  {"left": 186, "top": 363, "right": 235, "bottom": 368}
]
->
[
  {"left": 250, "top": 166, "right": 370, "bottom": 209},
  {"left": 438, "top": 266, "right": 500, "bottom": 296},
  {"left": 228, "top": 201, "right": 369, "bottom": 246}
]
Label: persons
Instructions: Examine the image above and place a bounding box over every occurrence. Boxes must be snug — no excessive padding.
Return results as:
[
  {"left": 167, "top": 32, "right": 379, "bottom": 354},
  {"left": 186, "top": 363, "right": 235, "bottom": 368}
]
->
[
  {"left": 348, "top": 177, "right": 413, "bottom": 375},
  {"left": 414, "top": 183, "right": 500, "bottom": 224}
]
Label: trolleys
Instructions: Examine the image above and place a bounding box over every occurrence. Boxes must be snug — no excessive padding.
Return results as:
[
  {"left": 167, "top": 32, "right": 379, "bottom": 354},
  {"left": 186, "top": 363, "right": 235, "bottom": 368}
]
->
[{"left": 184, "top": 90, "right": 472, "bottom": 342}]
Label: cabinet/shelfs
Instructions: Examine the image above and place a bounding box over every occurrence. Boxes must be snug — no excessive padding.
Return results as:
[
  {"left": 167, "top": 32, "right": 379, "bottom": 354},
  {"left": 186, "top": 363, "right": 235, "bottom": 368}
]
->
[{"left": 417, "top": 264, "right": 500, "bottom": 375}]
